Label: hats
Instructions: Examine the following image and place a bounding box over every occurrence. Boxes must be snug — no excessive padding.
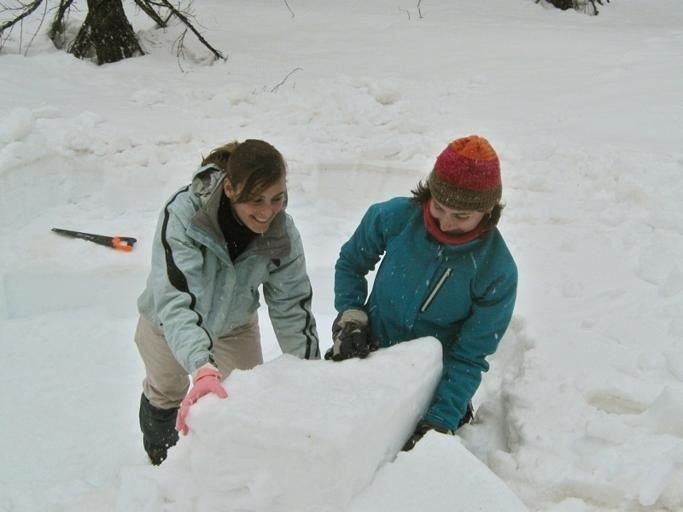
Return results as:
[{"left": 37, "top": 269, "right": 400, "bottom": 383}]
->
[{"left": 428, "top": 136, "right": 502, "bottom": 210}]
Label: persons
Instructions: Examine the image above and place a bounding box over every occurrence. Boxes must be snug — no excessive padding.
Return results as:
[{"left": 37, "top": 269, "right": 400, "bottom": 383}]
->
[
  {"left": 131, "top": 139, "right": 319, "bottom": 467},
  {"left": 322, "top": 134, "right": 521, "bottom": 454}
]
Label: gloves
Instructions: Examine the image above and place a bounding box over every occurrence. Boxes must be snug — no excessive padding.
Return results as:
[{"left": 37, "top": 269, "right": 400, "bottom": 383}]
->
[
  {"left": 401, "top": 421, "right": 455, "bottom": 451},
  {"left": 324, "top": 321, "right": 378, "bottom": 360},
  {"left": 175, "top": 367, "right": 228, "bottom": 436}
]
